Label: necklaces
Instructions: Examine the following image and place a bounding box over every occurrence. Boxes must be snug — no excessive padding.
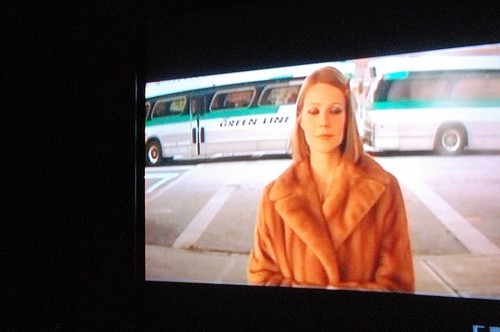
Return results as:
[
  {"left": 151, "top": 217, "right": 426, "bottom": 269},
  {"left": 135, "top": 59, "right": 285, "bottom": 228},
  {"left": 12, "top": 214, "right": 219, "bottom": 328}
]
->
[{"left": 307, "top": 158, "right": 342, "bottom": 204}]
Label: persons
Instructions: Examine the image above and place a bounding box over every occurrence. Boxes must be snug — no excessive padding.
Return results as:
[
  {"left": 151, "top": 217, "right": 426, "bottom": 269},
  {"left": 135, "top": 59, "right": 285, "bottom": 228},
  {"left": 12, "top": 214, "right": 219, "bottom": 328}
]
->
[{"left": 246, "top": 67, "right": 415, "bottom": 295}]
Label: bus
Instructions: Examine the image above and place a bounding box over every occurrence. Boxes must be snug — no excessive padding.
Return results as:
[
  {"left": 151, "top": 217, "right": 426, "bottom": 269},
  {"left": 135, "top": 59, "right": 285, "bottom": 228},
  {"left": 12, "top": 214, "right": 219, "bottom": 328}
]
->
[{"left": 144, "top": 52, "right": 500, "bottom": 166}]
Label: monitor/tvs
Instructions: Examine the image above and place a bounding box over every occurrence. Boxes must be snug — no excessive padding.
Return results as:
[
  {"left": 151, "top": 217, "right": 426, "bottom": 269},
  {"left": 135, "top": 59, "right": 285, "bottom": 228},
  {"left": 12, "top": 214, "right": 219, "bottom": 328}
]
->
[{"left": 129, "top": 32, "right": 500, "bottom": 331}]
